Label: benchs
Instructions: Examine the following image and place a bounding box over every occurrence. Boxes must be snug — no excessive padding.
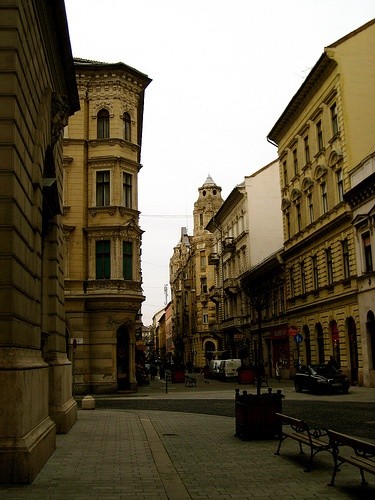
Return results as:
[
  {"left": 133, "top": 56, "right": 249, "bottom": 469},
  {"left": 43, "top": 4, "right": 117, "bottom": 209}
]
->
[
  {"left": 274, "top": 412, "right": 340, "bottom": 474},
  {"left": 327, "top": 430, "right": 375, "bottom": 487},
  {"left": 185, "top": 375, "right": 197, "bottom": 388}
]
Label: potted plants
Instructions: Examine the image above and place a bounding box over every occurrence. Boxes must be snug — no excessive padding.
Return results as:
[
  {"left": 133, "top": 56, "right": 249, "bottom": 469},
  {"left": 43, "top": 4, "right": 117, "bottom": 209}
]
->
[
  {"left": 204, "top": 365, "right": 210, "bottom": 380},
  {"left": 236, "top": 364, "right": 256, "bottom": 384},
  {"left": 174, "top": 362, "right": 186, "bottom": 383},
  {"left": 232, "top": 256, "right": 287, "bottom": 441}
]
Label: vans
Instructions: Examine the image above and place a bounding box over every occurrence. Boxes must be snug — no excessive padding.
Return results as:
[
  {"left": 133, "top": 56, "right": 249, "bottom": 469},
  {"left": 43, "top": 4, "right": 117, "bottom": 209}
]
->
[
  {"left": 219, "top": 359, "right": 241, "bottom": 381},
  {"left": 209, "top": 359, "right": 226, "bottom": 378}
]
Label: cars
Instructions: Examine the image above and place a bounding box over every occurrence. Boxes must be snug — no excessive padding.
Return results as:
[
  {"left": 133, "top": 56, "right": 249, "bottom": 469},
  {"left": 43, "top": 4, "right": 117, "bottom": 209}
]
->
[{"left": 294, "top": 363, "right": 350, "bottom": 394}]
